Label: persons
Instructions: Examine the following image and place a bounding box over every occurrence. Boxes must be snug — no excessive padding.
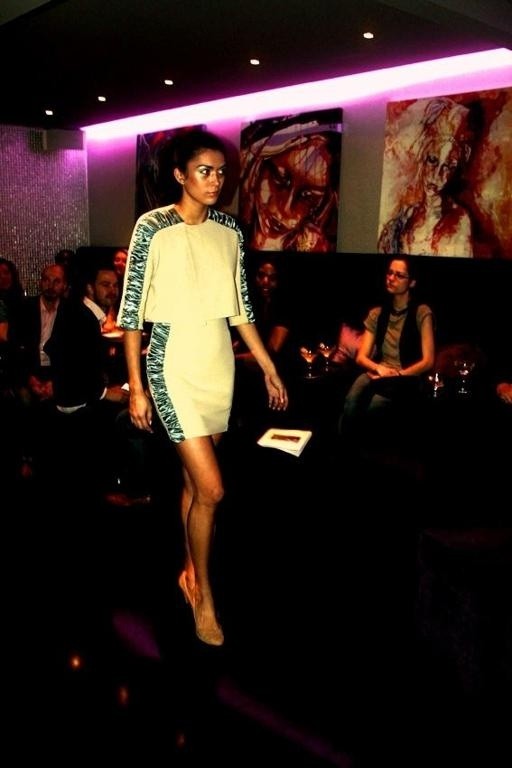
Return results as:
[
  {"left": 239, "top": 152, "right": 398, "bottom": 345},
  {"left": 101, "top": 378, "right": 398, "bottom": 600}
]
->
[
  {"left": 0, "top": 246, "right": 152, "bottom": 495},
  {"left": 242, "top": 119, "right": 344, "bottom": 251},
  {"left": 343, "top": 262, "right": 435, "bottom": 410},
  {"left": 115, "top": 126, "right": 295, "bottom": 658},
  {"left": 375, "top": 96, "right": 482, "bottom": 259},
  {"left": 231, "top": 251, "right": 291, "bottom": 371}
]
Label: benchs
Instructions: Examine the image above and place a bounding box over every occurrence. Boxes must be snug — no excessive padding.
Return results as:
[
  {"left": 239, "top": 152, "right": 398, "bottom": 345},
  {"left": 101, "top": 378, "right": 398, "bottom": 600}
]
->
[{"left": 72, "top": 244, "right": 512, "bottom": 475}]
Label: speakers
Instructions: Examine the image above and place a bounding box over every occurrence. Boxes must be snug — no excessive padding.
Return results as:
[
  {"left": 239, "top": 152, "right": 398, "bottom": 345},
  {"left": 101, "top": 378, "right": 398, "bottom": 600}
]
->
[{"left": 42, "top": 128, "right": 83, "bottom": 150}]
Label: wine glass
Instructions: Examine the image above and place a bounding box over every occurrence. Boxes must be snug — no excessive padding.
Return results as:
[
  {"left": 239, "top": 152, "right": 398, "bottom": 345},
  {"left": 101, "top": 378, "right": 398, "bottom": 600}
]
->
[
  {"left": 452, "top": 358, "right": 477, "bottom": 395},
  {"left": 297, "top": 343, "right": 321, "bottom": 379},
  {"left": 426, "top": 368, "right": 446, "bottom": 399},
  {"left": 318, "top": 340, "right": 338, "bottom": 376}
]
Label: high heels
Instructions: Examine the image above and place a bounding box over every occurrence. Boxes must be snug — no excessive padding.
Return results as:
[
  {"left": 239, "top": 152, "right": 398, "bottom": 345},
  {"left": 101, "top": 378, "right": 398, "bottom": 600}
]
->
[
  {"left": 191, "top": 582, "right": 225, "bottom": 646},
  {"left": 178, "top": 571, "right": 191, "bottom": 604}
]
